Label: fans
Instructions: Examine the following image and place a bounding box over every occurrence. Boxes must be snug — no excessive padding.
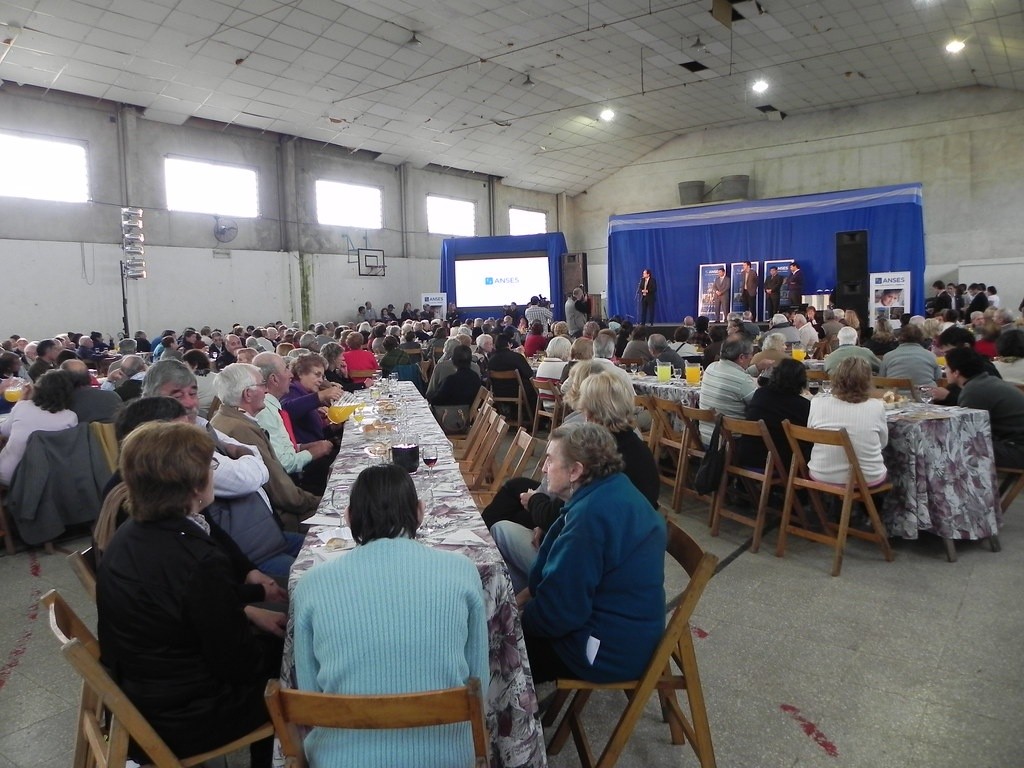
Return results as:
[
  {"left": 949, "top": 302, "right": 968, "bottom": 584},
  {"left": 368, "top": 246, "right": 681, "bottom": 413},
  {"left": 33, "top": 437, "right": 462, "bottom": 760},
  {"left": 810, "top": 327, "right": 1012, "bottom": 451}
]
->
[{"left": 214, "top": 217, "right": 238, "bottom": 243}]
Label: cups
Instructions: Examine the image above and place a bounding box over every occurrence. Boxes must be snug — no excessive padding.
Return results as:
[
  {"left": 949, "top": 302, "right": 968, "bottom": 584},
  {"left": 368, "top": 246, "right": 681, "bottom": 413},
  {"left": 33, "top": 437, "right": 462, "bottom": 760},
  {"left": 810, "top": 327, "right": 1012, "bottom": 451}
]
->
[
  {"left": 809, "top": 382, "right": 820, "bottom": 396},
  {"left": 88, "top": 369, "right": 99, "bottom": 378},
  {"left": 822, "top": 380, "right": 833, "bottom": 395},
  {"left": 104, "top": 347, "right": 108, "bottom": 351},
  {"left": 619, "top": 363, "right": 626, "bottom": 371},
  {"left": 95, "top": 348, "right": 99, "bottom": 353}
]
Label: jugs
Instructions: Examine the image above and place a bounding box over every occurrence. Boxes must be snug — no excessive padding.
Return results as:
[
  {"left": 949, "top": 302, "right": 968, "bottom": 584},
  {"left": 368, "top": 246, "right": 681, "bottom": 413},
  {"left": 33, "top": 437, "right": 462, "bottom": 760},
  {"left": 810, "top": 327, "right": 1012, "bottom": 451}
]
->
[
  {"left": 791, "top": 343, "right": 807, "bottom": 361},
  {"left": 931, "top": 343, "right": 945, "bottom": 365},
  {"left": 656, "top": 359, "right": 675, "bottom": 386},
  {"left": 757, "top": 363, "right": 777, "bottom": 386},
  {"left": 684, "top": 360, "right": 704, "bottom": 387},
  {"left": 327, "top": 387, "right": 360, "bottom": 424},
  {"left": 388, "top": 432, "right": 420, "bottom": 475},
  {"left": 3, "top": 377, "right": 26, "bottom": 403}
]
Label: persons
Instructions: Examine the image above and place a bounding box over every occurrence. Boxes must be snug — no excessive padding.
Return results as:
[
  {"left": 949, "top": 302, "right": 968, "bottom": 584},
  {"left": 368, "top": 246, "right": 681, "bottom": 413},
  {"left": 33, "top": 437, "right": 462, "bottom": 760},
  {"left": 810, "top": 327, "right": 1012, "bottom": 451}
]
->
[
  {"left": 713, "top": 268, "right": 731, "bottom": 323},
  {"left": 875, "top": 289, "right": 902, "bottom": 306},
  {"left": 638, "top": 269, "right": 657, "bottom": 326},
  {"left": 634, "top": 304, "right": 1024, "bottom": 533},
  {"left": 789, "top": 262, "right": 803, "bottom": 306},
  {"left": 357, "top": 301, "right": 397, "bottom": 323},
  {"left": 741, "top": 261, "right": 759, "bottom": 322},
  {"left": 924, "top": 279, "right": 998, "bottom": 325},
  {"left": 0, "top": 316, "right": 666, "bottom": 768},
  {"left": 400, "top": 302, "right": 434, "bottom": 324},
  {"left": 763, "top": 266, "right": 783, "bottom": 321},
  {"left": 503, "top": 288, "right": 589, "bottom": 340},
  {"left": 446, "top": 302, "right": 458, "bottom": 324}
]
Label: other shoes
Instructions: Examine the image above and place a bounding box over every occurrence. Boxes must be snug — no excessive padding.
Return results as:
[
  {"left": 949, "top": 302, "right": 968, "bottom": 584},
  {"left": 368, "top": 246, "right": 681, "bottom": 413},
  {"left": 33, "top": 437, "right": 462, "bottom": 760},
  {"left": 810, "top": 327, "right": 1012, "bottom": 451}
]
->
[
  {"left": 639, "top": 322, "right": 646, "bottom": 326},
  {"left": 713, "top": 320, "right": 721, "bottom": 324}
]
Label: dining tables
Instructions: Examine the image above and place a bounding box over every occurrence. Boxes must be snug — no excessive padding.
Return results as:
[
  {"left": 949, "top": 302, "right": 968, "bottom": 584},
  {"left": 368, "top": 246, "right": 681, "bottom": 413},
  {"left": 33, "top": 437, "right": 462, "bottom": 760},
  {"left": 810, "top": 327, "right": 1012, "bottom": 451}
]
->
[
  {"left": 628, "top": 372, "right": 1005, "bottom": 563},
  {"left": 272, "top": 381, "right": 548, "bottom": 768}
]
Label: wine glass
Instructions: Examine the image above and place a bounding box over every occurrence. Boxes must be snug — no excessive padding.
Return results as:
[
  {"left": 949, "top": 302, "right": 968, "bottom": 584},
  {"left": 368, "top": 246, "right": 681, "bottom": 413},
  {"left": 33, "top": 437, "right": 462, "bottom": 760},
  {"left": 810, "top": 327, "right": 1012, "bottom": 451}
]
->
[
  {"left": 807, "top": 349, "right": 814, "bottom": 360},
  {"left": 331, "top": 485, "right": 351, "bottom": 531},
  {"left": 673, "top": 368, "right": 682, "bottom": 385},
  {"left": 213, "top": 352, "right": 218, "bottom": 359},
  {"left": 920, "top": 387, "right": 933, "bottom": 412},
  {"left": 630, "top": 362, "right": 638, "bottom": 380},
  {"left": 353, "top": 369, "right": 440, "bottom": 534}
]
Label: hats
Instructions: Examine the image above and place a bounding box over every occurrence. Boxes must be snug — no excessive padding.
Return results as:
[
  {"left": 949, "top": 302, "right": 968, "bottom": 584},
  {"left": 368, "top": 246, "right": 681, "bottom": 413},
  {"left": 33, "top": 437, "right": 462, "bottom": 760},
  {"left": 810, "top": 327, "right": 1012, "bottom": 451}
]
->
[{"left": 16, "top": 338, "right": 29, "bottom": 345}]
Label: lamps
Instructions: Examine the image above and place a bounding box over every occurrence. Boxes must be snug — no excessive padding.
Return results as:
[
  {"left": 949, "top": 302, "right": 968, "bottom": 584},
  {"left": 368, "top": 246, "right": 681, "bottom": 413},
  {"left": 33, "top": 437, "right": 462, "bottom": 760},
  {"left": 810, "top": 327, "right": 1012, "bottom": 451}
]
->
[
  {"left": 405, "top": 32, "right": 424, "bottom": 49},
  {"left": 522, "top": 74, "right": 537, "bottom": 89},
  {"left": 690, "top": 37, "right": 707, "bottom": 51}
]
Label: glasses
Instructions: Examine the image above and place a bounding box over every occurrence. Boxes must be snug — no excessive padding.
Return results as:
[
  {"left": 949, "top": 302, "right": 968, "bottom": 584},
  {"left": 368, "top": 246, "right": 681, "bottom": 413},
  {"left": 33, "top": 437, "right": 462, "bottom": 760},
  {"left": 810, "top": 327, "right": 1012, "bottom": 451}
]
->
[
  {"left": 206, "top": 458, "right": 220, "bottom": 472},
  {"left": 241, "top": 380, "right": 267, "bottom": 400},
  {"left": 311, "top": 368, "right": 326, "bottom": 381}
]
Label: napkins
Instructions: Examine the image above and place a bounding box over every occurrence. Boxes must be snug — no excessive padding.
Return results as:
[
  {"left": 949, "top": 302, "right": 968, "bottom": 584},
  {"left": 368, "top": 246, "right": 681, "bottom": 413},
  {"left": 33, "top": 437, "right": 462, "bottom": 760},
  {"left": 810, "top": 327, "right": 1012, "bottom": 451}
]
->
[
  {"left": 329, "top": 474, "right": 359, "bottom": 481},
  {"left": 440, "top": 528, "right": 490, "bottom": 547},
  {"left": 315, "top": 527, "right": 357, "bottom": 544},
  {"left": 425, "top": 462, "right": 460, "bottom": 471},
  {"left": 886, "top": 409, "right": 913, "bottom": 416},
  {"left": 428, "top": 483, "right": 463, "bottom": 497},
  {"left": 300, "top": 513, "right": 345, "bottom": 526}
]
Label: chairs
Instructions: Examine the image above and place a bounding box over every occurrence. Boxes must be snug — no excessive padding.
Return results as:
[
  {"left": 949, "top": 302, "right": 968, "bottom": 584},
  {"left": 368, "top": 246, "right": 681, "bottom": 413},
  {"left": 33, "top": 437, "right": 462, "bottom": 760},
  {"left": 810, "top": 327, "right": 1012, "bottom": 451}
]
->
[{"left": 0, "top": 332, "right": 1024, "bottom": 768}]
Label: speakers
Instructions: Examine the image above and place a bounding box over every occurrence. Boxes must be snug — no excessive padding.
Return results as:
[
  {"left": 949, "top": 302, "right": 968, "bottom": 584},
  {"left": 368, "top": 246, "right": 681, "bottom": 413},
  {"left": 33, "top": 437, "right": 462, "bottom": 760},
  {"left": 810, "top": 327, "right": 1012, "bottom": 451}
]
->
[{"left": 836, "top": 228, "right": 869, "bottom": 332}]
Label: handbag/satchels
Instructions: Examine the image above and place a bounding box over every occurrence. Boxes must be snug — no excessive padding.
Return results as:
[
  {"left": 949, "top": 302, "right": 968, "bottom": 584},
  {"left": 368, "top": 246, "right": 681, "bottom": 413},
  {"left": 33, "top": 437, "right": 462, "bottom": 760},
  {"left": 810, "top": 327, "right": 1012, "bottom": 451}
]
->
[
  {"left": 692, "top": 413, "right": 727, "bottom": 496},
  {"left": 435, "top": 405, "right": 471, "bottom": 435}
]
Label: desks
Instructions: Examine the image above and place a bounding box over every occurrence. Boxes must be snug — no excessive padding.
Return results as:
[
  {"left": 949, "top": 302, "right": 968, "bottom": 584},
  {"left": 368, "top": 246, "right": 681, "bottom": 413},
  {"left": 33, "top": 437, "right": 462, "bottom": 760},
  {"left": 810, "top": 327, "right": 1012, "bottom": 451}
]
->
[{"left": 800, "top": 291, "right": 832, "bottom": 311}]
